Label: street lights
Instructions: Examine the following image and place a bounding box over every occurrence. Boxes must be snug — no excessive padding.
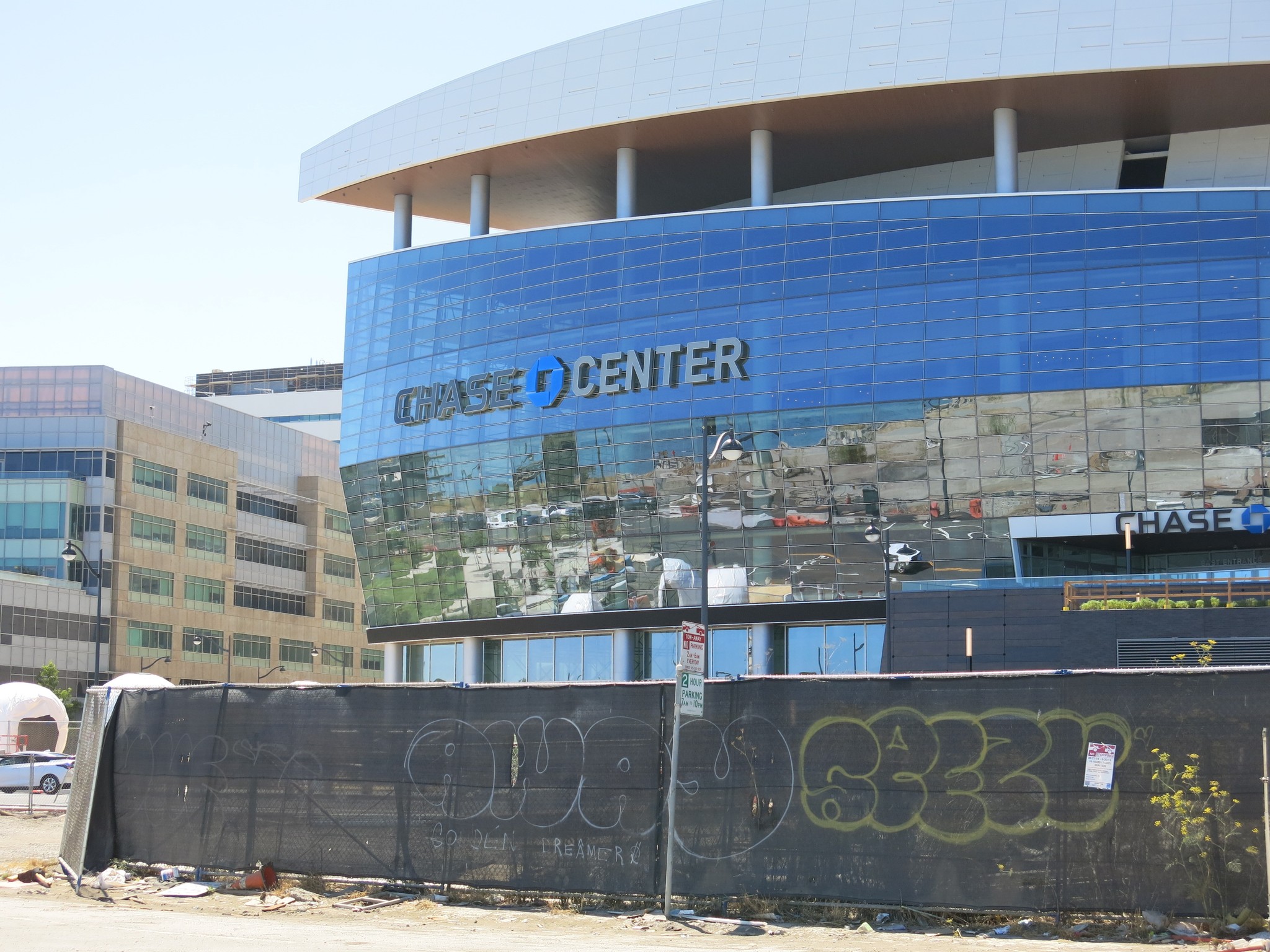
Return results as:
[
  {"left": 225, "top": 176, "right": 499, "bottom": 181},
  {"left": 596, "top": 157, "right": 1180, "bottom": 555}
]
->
[
  {"left": 311, "top": 646, "right": 349, "bottom": 686},
  {"left": 59, "top": 541, "right": 103, "bottom": 688},
  {"left": 699, "top": 423, "right": 744, "bottom": 684},
  {"left": 258, "top": 665, "right": 286, "bottom": 684},
  {"left": 192, "top": 635, "right": 234, "bottom": 685},
  {"left": 140, "top": 656, "right": 172, "bottom": 674}
]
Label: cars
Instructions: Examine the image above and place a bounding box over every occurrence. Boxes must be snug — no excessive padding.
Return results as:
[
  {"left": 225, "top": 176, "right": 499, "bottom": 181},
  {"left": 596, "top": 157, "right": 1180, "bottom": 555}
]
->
[
  {"left": 413, "top": 481, "right": 886, "bottom": 527},
  {"left": 0, "top": 749, "right": 77, "bottom": 794}
]
